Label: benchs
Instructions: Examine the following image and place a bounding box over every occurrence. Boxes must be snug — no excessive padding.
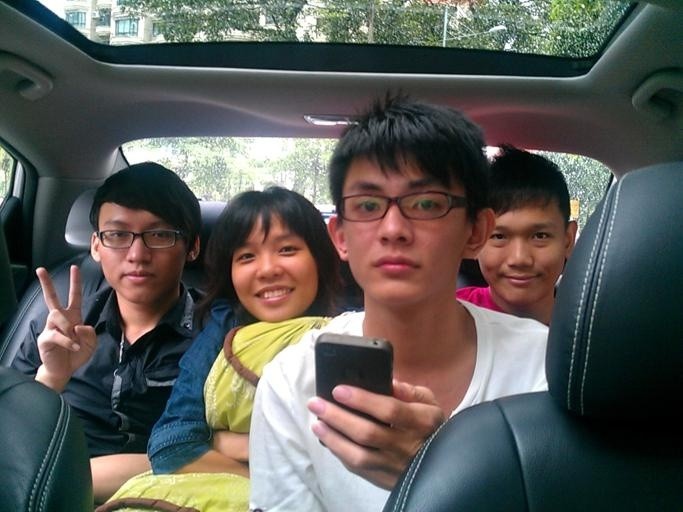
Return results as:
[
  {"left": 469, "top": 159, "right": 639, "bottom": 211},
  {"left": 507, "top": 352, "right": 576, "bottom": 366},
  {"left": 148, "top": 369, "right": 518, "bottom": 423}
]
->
[{"left": 1, "top": 189, "right": 364, "bottom": 377}]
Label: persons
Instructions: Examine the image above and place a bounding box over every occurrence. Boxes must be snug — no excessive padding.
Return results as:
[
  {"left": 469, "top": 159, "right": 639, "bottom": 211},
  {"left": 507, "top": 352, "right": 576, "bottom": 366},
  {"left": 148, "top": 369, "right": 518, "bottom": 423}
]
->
[
  {"left": 451, "top": 138, "right": 577, "bottom": 323},
  {"left": 146, "top": 185, "right": 363, "bottom": 482},
  {"left": 10, "top": 163, "right": 216, "bottom": 511},
  {"left": 246, "top": 87, "right": 553, "bottom": 512}
]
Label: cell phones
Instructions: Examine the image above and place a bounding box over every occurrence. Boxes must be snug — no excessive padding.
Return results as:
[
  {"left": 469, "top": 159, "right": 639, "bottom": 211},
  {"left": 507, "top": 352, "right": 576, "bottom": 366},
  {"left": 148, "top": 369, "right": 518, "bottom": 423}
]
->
[{"left": 314, "top": 333, "right": 394, "bottom": 452}]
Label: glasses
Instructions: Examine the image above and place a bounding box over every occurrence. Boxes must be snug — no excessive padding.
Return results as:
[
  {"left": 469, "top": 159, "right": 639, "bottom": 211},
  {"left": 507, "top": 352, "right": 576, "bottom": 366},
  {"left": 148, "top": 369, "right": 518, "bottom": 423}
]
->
[
  {"left": 96, "top": 227, "right": 186, "bottom": 249},
  {"left": 334, "top": 190, "right": 474, "bottom": 222}
]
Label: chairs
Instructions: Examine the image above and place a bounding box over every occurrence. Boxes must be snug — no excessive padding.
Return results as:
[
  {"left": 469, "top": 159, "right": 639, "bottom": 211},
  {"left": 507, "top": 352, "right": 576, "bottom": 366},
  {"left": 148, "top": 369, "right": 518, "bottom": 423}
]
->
[
  {"left": 0, "top": 367, "right": 95, "bottom": 511},
  {"left": 371, "top": 161, "right": 682, "bottom": 512}
]
